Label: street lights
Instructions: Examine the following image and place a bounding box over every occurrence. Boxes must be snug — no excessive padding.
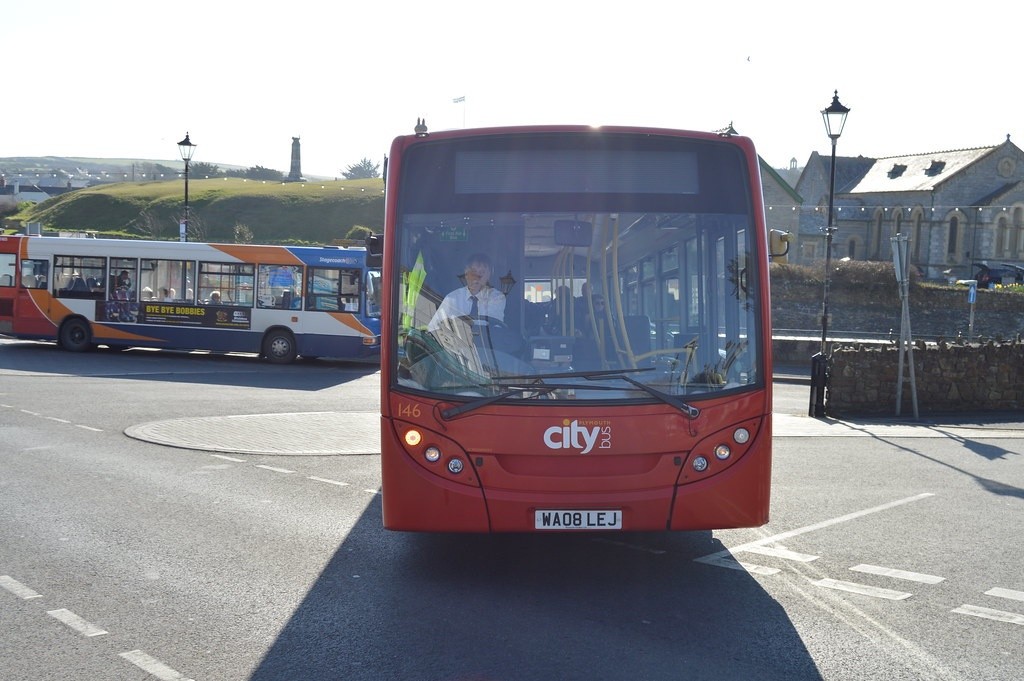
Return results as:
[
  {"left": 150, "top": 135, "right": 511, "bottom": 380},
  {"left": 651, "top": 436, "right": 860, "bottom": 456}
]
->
[
  {"left": 177, "top": 131, "right": 196, "bottom": 242},
  {"left": 809, "top": 89, "right": 852, "bottom": 418}
]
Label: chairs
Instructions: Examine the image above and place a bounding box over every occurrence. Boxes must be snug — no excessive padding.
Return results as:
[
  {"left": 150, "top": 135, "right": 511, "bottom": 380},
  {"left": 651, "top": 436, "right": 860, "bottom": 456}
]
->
[
  {"left": 618, "top": 314, "right": 652, "bottom": 366},
  {"left": 0, "top": 274, "right": 104, "bottom": 292}
]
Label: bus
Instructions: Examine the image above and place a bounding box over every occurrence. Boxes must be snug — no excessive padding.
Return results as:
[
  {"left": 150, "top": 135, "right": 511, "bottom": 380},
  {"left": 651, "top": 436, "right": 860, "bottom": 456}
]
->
[
  {"left": 0, "top": 236, "right": 382, "bottom": 364},
  {"left": 365, "top": 117, "right": 794, "bottom": 534}
]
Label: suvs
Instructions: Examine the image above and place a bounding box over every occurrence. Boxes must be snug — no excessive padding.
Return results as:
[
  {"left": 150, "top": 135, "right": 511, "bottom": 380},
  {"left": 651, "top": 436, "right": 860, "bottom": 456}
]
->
[{"left": 973, "top": 260, "right": 1024, "bottom": 289}]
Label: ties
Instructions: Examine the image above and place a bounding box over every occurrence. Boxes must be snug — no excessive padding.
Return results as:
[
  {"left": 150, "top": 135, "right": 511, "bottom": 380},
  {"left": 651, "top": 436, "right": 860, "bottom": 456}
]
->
[{"left": 469, "top": 296, "right": 479, "bottom": 315}]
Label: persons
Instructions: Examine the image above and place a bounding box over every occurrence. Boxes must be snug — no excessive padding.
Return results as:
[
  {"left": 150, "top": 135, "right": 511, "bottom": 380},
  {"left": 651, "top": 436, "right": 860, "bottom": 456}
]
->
[
  {"left": 208, "top": 291, "right": 223, "bottom": 305},
  {"left": 548, "top": 283, "right": 617, "bottom": 336},
  {"left": 117, "top": 270, "right": 132, "bottom": 290},
  {"left": 142, "top": 287, "right": 153, "bottom": 301},
  {"left": 164, "top": 288, "right": 176, "bottom": 302},
  {"left": 39, "top": 256, "right": 58, "bottom": 289},
  {"left": 427, "top": 253, "right": 506, "bottom": 334}
]
